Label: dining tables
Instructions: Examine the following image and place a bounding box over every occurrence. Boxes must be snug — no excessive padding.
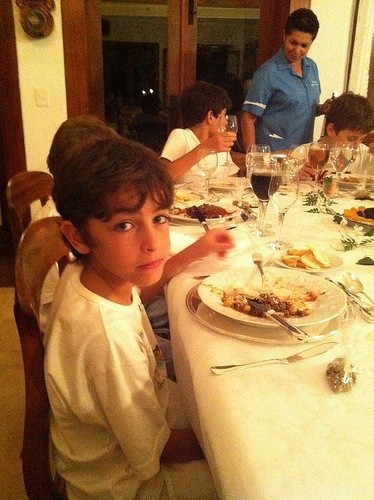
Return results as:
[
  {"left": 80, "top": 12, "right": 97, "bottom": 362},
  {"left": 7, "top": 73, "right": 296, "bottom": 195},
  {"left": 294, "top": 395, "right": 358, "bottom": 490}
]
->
[{"left": 163, "top": 177, "right": 374, "bottom": 500}]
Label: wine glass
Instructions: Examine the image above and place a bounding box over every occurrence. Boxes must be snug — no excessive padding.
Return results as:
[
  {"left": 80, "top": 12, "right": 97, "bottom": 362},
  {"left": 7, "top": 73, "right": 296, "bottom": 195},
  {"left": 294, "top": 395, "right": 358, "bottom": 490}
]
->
[
  {"left": 308, "top": 142, "right": 354, "bottom": 214},
  {"left": 197, "top": 148, "right": 218, "bottom": 198},
  {"left": 218, "top": 115, "right": 238, "bottom": 167},
  {"left": 245, "top": 143, "right": 307, "bottom": 252}
]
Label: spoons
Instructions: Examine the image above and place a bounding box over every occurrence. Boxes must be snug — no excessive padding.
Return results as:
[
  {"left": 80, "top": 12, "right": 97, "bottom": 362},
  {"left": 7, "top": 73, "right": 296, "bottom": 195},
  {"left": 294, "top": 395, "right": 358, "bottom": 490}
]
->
[{"left": 344, "top": 272, "right": 374, "bottom": 304}]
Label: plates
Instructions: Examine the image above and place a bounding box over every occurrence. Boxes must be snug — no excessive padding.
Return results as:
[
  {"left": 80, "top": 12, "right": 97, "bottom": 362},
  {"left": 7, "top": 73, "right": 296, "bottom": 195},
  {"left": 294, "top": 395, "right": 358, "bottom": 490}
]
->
[
  {"left": 168, "top": 201, "right": 240, "bottom": 223},
  {"left": 208, "top": 177, "right": 250, "bottom": 191},
  {"left": 271, "top": 249, "right": 344, "bottom": 272},
  {"left": 186, "top": 266, "right": 355, "bottom": 344}
]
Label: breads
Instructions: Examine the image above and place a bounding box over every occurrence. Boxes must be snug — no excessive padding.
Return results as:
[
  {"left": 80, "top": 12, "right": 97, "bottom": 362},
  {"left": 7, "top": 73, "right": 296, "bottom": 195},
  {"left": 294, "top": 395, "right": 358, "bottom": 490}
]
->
[{"left": 282, "top": 244, "right": 330, "bottom": 269}]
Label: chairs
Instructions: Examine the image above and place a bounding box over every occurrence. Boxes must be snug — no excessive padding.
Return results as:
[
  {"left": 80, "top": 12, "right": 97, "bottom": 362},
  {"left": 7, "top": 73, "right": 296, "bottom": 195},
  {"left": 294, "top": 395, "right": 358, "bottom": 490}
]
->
[
  {"left": 12, "top": 216, "right": 75, "bottom": 500},
  {"left": 7, "top": 171, "right": 54, "bottom": 248}
]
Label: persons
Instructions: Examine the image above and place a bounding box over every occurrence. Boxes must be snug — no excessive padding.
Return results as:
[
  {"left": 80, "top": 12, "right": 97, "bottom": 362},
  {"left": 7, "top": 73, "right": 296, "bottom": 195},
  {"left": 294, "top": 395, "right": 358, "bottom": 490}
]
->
[
  {"left": 290, "top": 91, "right": 374, "bottom": 180},
  {"left": 161, "top": 83, "right": 240, "bottom": 184},
  {"left": 43, "top": 137, "right": 235, "bottom": 500},
  {"left": 240, "top": 8, "right": 334, "bottom": 154},
  {"left": 15, "top": 115, "right": 176, "bottom": 381}
]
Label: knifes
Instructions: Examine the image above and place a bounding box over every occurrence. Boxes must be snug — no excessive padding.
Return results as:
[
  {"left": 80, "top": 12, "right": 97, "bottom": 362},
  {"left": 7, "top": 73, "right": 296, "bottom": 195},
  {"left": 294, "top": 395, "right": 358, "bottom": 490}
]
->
[
  {"left": 210, "top": 342, "right": 338, "bottom": 375},
  {"left": 248, "top": 297, "right": 309, "bottom": 343},
  {"left": 193, "top": 205, "right": 210, "bottom": 232}
]
